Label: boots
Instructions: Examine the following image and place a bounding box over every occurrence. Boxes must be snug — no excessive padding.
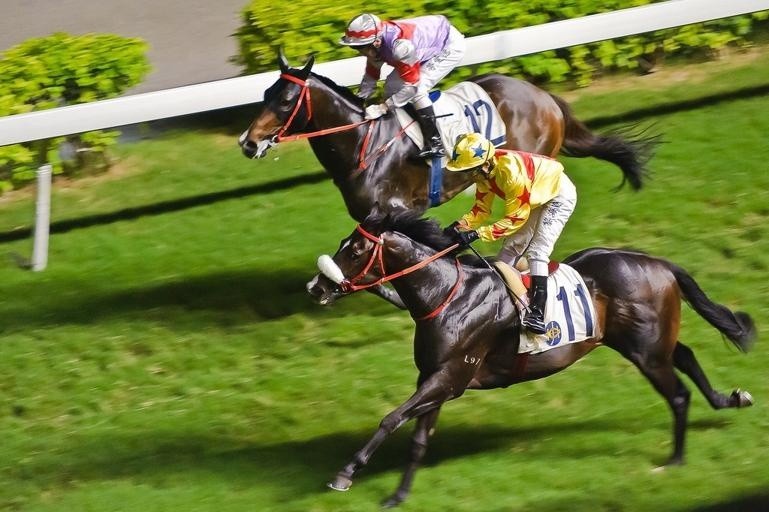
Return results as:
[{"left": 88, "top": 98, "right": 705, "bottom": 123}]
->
[
  {"left": 415, "top": 103, "right": 446, "bottom": 158},
  {"left": 524, "top": 274, "right": 548, "bottom": 334}
]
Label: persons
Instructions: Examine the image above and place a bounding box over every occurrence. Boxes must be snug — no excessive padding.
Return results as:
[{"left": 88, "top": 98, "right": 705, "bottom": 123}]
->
[
  {"left": 337, "top": 12, "right": 466, "bottom": 163},
  {"left": 441, "top": 129, "right": 577, "bottom": 336}
]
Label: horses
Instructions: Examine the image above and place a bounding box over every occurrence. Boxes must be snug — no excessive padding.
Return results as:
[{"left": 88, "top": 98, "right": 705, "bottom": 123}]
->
[
  {"left": 303, "top": 201, "right": 757, "bottom": 511},
  {"left": 236, "top": 44, "right": 673, "bottom": 311}
]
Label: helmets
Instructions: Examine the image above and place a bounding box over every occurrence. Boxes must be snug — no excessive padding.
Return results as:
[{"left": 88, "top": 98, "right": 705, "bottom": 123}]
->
[
  {"left": 444, "top": 131, "right": 497, "bottom": 173},
  {"left": 338, "top": 12, "right": 387, "bottom": 48}
]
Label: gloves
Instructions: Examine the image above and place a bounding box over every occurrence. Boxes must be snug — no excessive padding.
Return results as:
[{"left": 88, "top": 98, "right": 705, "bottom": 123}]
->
[
  {"left": 454, "top": 229, "right": 480, "bottom": 247},
  {"left": 363, "top": 102, "right": 388, "bottom": 121},
  {"left": 443, "top": 220, "right": 462, "bottom": 236}
]
